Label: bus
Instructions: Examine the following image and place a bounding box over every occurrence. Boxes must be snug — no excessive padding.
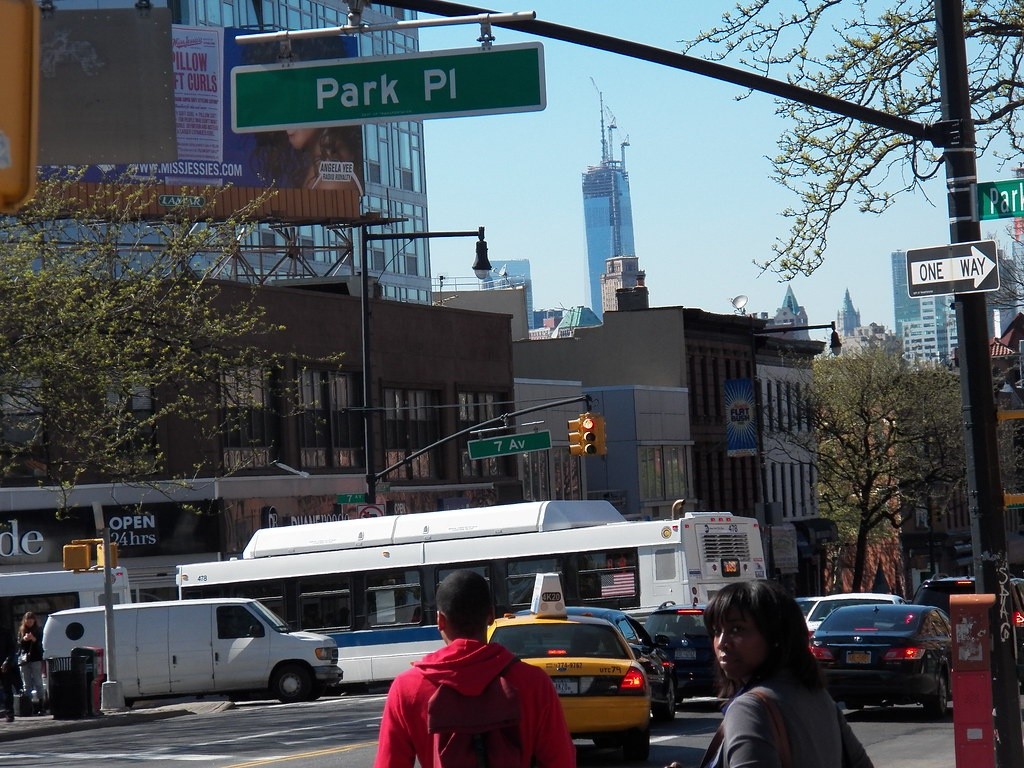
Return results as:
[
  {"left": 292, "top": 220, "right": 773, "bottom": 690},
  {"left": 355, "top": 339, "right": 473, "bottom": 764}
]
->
[
  {"left": 173, "top": 501, "right": 767, "bottom": 686},
  {"left": 0, "top": 565, "right": 133, "bottom": 688}
]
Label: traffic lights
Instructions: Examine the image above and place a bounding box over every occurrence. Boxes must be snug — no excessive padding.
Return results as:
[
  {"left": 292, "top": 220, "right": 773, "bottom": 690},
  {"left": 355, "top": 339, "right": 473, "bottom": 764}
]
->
[{"left": 579, "top": 416, "right": 598, "bottom": 456}]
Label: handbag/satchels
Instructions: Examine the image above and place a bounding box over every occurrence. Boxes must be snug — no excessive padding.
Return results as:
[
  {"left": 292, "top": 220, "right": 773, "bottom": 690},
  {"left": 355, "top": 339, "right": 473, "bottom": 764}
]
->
[{"left": 18, "top": 652, "right": 32, "bottom": 666}]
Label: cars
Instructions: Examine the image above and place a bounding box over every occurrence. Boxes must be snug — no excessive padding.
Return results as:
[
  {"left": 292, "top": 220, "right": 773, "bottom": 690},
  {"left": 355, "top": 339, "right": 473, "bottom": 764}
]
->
[
  {"left": 789, "top": 571, "right": 1024, "bottom": 719},
  {"left": 486, "top": 573, "right": 653, "bottom": 766},
  {"left": 514, "top": 605, "right": 678, "bottom": 728}
]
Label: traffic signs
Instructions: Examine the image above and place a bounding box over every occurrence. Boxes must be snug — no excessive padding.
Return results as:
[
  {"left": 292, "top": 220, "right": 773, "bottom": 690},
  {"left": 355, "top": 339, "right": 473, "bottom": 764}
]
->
[
  {"left": 905, "top": 239, "right": 1001, "bottom": 302},
  {"left": 566, "top": 418, "right": 582, "bottom": 456}
]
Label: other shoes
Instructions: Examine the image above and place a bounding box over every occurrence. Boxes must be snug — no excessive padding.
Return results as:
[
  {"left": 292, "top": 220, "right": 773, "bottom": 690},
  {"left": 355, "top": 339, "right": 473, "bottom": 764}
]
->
[
  {"left": 6, "top": 714, "right": 14, "bottom": 723},
  {"left": 39, "top": 709, "right": 46, "bottom": 716}
]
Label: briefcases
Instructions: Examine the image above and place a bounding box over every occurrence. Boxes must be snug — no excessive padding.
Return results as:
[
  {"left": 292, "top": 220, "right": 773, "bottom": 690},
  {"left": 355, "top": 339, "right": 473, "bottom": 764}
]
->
[{"left": 13, "top": 689, "right": 32, "bottom": 716}]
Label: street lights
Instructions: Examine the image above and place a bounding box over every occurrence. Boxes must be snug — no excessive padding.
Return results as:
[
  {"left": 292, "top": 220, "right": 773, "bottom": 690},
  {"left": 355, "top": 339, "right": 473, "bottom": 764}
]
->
[
  {"left": 751, "top": 319, "right": 843, "bottom": 578},
  {"left": 357, "top": 222, "right": 495, "bottom": 507}
]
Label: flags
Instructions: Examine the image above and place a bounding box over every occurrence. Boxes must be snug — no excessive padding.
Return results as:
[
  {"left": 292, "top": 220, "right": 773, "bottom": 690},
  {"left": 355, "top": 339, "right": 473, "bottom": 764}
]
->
[{"left": 601, "top": 572, "right": 635, "bottom": 596}]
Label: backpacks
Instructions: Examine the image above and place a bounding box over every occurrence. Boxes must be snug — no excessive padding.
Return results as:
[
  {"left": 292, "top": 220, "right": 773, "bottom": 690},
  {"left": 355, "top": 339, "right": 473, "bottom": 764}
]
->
[{"left": 428, "top": 656, "right": 524, "bottom": 768}]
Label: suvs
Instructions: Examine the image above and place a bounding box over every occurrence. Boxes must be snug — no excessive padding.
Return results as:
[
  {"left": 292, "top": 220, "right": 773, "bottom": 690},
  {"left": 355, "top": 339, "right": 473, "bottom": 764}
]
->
[{"left": 642, "top": 599, "right": 724, "bottom": 704}]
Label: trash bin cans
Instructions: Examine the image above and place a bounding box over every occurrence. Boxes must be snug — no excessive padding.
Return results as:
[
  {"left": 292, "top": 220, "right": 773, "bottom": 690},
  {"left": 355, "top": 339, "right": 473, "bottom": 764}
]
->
[{"left": 46, "top": 656, "right": 89, "bottom": 720}]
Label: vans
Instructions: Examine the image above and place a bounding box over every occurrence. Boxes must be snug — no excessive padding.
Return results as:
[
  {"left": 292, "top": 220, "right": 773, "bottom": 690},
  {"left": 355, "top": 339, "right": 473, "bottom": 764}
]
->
[{"left": 36, "top": 596, "right": 346, "bottom": 705}]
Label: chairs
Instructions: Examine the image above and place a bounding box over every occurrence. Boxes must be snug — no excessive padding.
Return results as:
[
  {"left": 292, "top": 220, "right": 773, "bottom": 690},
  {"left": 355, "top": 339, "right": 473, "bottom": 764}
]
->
[{"left": 569, "top": 628, "right": 600, "bottom": 655}]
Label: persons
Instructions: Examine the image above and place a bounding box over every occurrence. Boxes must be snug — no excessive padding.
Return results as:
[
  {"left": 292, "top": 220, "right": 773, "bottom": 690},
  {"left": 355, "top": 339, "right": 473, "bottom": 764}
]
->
[
  {"left": 245, "top": 34, "right": 364, "bottom": 196},
  {"left": 665, "top": 579, "right": 874, "bottom": 768},
  {"left": 17, "top": 612, "right": 46, "bottom": 716},
  {"left": 374, "top": 569, "right": 577, "bottom": 768},
  {"left": 339, "top": 607, "right": 349, "bottom": 626},
  {"left": 0, "top": 626, "right": 16, "bottom": 723}
]
